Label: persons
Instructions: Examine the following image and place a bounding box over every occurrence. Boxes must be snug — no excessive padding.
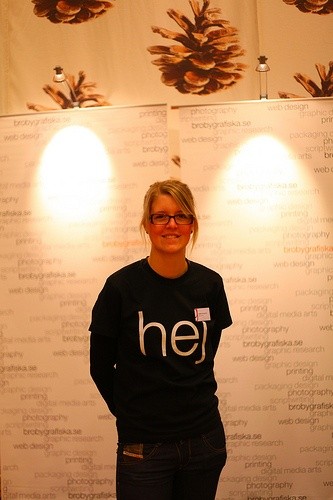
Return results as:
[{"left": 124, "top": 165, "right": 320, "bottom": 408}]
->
[{"left": 88, "top": 179, "right": 233, "bottom": 500}]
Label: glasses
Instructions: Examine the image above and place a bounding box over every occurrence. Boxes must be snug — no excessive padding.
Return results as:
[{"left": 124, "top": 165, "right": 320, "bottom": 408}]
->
[{"left": 149, "top": 213, "right": 193, "bottom": 225}]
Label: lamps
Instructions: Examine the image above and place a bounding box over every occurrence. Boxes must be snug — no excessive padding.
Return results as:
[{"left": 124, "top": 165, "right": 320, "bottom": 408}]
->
[
  {"left": 255, "top": 56, "right": 271, "bottom": 100},
  {"left": 52, "top": 65, "right": 80, "bottom": 109}
]
